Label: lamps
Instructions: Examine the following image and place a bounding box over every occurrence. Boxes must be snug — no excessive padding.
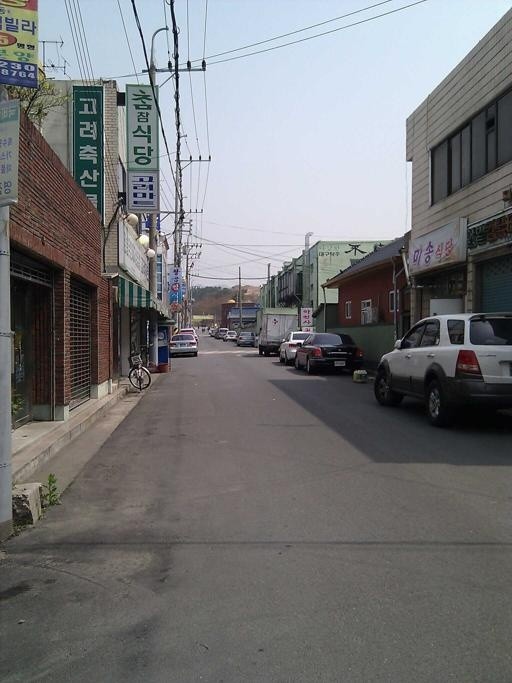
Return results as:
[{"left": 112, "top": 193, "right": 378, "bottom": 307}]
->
[{"left": 124, "top": 213, "right": 157, "bottom": 259}]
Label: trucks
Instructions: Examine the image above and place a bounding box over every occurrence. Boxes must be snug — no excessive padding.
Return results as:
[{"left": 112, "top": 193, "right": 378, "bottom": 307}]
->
[{"left": 258, "top": 313, "right": 299, "bottom": 357}]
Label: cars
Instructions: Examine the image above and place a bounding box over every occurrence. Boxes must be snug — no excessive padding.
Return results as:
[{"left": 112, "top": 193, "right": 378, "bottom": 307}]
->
[
  {"left": 278, "top": 331, "right": 363, "bottom": 374},
  {"left": 209, "top": 328, "right": 255, "bottom": 347},
  {"left": 374, "top": 311, "right": 512, "bottom": 426},
  {"left": 169, "top": 328, "right": 199, "bottom": 358}
]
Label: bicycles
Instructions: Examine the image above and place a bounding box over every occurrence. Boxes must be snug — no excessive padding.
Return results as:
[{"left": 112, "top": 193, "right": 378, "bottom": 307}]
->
[{"left": 128, "top": 353, "right": 152, "bottom": 391}]
